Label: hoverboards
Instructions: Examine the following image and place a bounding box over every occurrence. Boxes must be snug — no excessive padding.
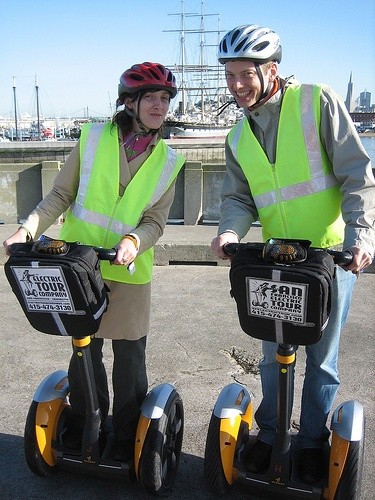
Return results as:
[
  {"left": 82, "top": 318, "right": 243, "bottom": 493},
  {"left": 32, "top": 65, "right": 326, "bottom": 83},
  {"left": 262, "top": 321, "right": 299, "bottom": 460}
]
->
[
  {"left": 201, "top": 235, "right": 365, "bottom": 500},
  {"left": 6, "top": 238, "right": 185, "bottom": 498}
]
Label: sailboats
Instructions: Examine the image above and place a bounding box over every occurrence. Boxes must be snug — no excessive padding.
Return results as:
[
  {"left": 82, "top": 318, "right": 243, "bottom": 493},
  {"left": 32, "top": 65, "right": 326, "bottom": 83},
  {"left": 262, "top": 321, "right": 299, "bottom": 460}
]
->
[{"left": 160, "top": 1, "right": 246, "bottom": 139}]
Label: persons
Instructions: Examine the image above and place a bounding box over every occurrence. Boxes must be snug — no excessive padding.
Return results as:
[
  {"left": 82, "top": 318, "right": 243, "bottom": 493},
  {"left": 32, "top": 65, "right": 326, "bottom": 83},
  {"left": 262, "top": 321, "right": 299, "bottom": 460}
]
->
[
  {"left": 4, "top": 62, "right": 186, "bottom": 465},
  {"left": 211, "top": 24, "right": 375, "bottom": 485}
]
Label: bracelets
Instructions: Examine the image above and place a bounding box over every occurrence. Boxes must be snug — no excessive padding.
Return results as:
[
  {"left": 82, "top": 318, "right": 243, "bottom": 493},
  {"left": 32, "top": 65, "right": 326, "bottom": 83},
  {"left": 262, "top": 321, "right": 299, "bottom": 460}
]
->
[{"left": 120, "top": 235, "right": 137, "bottom": 248}]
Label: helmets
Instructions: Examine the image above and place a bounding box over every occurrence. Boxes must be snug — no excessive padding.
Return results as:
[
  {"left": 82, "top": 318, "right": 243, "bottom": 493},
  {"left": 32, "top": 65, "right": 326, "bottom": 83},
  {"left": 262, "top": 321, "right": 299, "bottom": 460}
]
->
[
  {"left": 217, "top": 24, "right": 282, "bottom": 64},
  {"left": 118, "top": 62, "right": 177, "bottom": 98}
]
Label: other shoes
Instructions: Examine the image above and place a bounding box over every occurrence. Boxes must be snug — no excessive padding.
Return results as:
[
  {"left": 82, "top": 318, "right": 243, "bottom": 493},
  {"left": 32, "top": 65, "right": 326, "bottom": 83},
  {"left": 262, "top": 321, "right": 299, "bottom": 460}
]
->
[
  {"left": 61, "top": 415, "right": 87, "bottom": 451},
  {"left": 244, "top": 440, "right": 273, "bottom": 474},
  {"left": 295, "top": 441, "right": 329, "bottom": 482},
  {"left": 101, "top": 436, "right": 133, "bottom": 466}
]
